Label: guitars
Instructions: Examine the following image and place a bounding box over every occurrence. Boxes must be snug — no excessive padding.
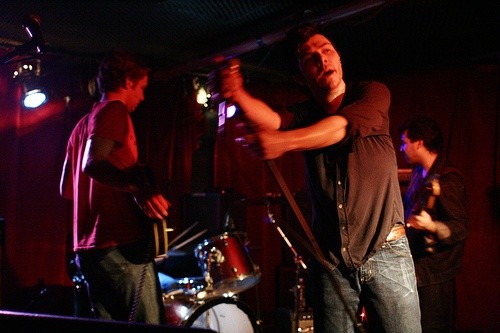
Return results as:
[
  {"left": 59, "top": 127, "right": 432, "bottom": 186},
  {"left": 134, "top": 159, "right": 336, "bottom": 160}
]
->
[
  {"left": 133, "top": 163, "right": 169, "bottom": 258},
  {"left": 404, "top": 176, "right": 441, "bottom": 252}
]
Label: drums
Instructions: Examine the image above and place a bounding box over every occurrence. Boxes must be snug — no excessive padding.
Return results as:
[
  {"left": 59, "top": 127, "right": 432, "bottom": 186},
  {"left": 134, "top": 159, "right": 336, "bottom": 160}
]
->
[
  {"left": 192, "top": 229, "right": 261, "bottom": 296},
  {"left": 160, "top": 286, "right": 264, "bottom": 333}
]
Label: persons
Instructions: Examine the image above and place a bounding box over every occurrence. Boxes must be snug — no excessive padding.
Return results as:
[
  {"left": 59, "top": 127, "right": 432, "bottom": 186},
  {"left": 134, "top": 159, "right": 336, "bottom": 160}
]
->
[
  {"left": 60, "top": 56, "right": 171, "bottom": 327},
  {"left": 398, "top": 117, "right": 469, "bottom": 332},
  {"left": 213, "top": 29, "right": 423, "bottom": 332}
]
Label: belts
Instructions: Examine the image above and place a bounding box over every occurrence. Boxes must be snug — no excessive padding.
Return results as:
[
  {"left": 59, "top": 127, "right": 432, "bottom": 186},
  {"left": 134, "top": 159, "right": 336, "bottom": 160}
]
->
[{"left": 384, "top": 224, "right": 406, "bottom": 242}]
